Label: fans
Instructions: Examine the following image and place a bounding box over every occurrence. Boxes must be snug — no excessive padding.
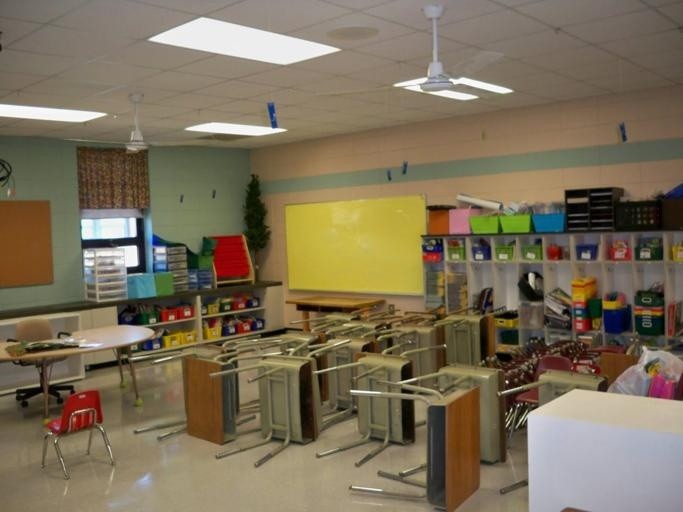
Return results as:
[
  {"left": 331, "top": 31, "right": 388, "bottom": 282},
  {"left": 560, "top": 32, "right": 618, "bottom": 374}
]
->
[
  {"left": 313, "top": 4, "right": 518, "bottom": 104},
  {"left": 61, "top": 88, "right": 213, "bottom": 154}
]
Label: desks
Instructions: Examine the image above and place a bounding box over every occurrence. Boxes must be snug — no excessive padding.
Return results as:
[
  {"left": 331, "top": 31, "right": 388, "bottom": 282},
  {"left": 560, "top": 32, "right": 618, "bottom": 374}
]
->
[
  {"left": 346, "top": 381, "right": 481, "bottom": 511},
  {"left": 496, "top": 368, "right": 610, "bottom": 493},
  {"left": 595, "top": 343, "right": 639, "bottom": 388},
  {"left": 0, "top": 325, "right": 156, "bottom": 425},
  {"left": 133, "top": 296, "right": 506, "bottom": 468}
]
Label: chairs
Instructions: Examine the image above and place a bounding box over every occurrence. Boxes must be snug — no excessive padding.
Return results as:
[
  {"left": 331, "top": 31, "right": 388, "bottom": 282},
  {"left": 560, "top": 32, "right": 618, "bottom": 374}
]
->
[
  {"left": 5, "top": 316, "right": 75, "bottom": 407},
  {"left": 39, "top": 388, "right": 115, "bottom": 481}
]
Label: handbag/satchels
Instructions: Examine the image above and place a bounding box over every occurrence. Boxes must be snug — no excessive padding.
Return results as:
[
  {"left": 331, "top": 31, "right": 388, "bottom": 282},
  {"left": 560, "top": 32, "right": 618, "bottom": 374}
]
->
[{"left": 646, "top": 356, "right": 682, "bottom": 399}]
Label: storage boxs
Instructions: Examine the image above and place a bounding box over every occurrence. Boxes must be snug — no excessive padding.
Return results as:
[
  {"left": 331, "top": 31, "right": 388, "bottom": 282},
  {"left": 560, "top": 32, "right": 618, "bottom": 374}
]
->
[
  {"left": 421, "top": 185, "right": 682, "bottom": 262},
  {"left": 125, "top": 272, "right": 156, "bottom": 300},
  {"left": 152, "top": 271, "right": 174, "bottom": 298}
]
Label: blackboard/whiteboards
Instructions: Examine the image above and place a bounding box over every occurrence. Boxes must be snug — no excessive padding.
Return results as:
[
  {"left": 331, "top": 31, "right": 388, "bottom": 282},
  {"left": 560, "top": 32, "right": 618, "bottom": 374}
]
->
[{"left": 284, "top": 194, "right": 428, "bottom": 297}]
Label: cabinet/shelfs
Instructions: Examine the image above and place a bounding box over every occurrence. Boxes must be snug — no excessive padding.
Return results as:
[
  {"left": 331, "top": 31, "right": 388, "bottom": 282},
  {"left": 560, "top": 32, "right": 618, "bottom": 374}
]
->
[
  {"left": 81, "top": 247, "right": 127, "bottom": 303},
  {"left": 152, "top": 244, "right": 188, "bottom": 292},
  {"left": 120, "top": 279, "right": 282, "bottom": 360},
  {"left": 0, "top": 299, "right": 121, "bottom": 397},
  {"left": 422, "top": 230, "right": 683, "bottom": 356}
]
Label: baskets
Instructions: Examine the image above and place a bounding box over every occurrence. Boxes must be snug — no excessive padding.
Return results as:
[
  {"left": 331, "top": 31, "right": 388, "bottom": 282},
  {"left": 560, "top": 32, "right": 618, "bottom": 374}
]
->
[{"left": 614, "top": 201, "right": 662, "bottom": 232}]
